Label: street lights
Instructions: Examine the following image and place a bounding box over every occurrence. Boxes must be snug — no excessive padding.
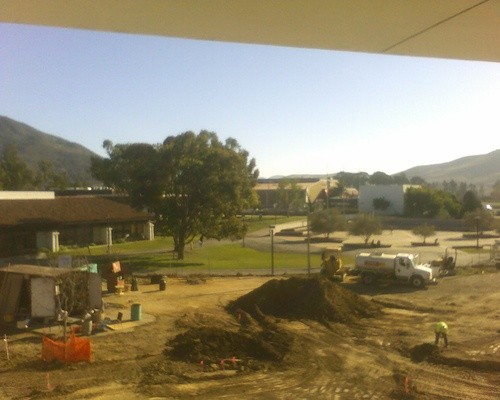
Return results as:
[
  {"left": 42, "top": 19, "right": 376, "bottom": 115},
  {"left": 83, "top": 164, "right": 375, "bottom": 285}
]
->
[
  {"left": 269, "top": 225, "right": 276, "bottom": 274},
  {"left": 475, "top": 216, "right": 481, "bottom": 248}
]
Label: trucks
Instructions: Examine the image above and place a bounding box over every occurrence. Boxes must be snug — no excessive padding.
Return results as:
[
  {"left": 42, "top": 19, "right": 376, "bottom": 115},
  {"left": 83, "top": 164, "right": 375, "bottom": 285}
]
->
[{"left": 351, "top": 252, "right": 433, "bottom": 288}]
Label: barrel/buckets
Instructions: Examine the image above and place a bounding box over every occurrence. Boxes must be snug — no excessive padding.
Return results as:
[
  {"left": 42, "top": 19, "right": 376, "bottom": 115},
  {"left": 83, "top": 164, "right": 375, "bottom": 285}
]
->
[
  {"left": 160, "top": 279, "right": 166, "bottom": 290},
  {"left": 82, "top": 319, "right": 92, "bottom": 336},
  {"left": 131, "top": 303, "right": 141, "bottom": 320}
]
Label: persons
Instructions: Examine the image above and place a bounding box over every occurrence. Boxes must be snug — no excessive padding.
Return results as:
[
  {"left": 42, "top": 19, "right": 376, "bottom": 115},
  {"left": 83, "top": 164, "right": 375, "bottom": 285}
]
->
[{"left": 433, "top": 321, "right": 450, "bottom": 352}]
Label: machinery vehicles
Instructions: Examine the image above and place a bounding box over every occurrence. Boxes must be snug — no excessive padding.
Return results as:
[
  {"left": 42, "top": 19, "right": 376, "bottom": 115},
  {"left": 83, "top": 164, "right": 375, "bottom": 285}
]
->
[{"left": 319, "top": 246, "right": 351, "bottom": 281}]
